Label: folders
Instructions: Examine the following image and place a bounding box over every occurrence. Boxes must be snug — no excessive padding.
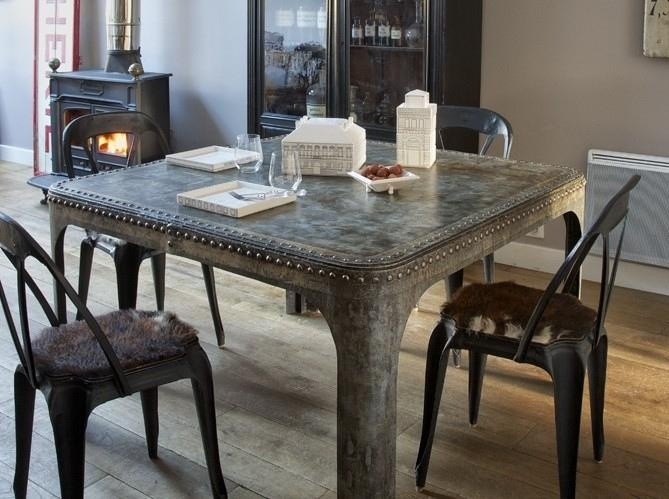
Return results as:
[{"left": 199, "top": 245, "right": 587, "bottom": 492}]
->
[{"left": 584, "top": 149, "right": 669, "bottom": 269}]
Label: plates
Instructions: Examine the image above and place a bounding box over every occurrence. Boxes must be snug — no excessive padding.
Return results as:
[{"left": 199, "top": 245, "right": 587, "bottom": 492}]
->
[{"left": 247, "top": 1, "right": 483, "bottom": 154}]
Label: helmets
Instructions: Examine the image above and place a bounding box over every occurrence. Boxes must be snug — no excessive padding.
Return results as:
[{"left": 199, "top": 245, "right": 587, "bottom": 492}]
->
[
  {"left": 165, "top": 145, "right": 260, "bottom": 173},
  {"left": 176, "top": 181, "right": 296, "bottom": 217},
  {"left": 347, "top": 166, "right": 420, "bottom": 192}
]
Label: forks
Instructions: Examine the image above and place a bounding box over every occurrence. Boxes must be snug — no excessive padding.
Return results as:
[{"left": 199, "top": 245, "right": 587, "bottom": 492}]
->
[{"left": 229, "top": 190, "right": 264, "bottom": 200}]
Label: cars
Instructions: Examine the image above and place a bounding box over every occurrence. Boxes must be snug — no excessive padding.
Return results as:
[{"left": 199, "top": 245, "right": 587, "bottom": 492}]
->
[
  {"left": 269, "top": 152, "right": 301, "bottom": 195},
  {"left": 235, "top": 135, "right": 262, "bottom": 172}
]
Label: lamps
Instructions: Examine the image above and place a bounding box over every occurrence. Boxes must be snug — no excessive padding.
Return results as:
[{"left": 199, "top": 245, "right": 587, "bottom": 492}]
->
[
  {"left": 412, "top": 174, "right": 641, "bottom": 499},
  {"left": 0, "top": 211, "right": 228, "bottom": 499},
  {"left": 60, "top": 111, "right": 225, "bottom": 347},
  {"left": 433, "top": 105, "right": 514, "bottom": 285}
]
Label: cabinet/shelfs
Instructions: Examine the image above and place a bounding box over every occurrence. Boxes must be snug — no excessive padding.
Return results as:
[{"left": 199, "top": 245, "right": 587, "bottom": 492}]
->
[
  {"left": 351, "top": 16, "right": 362, "bottom": 46},
  {"left": 364, "top": 10, "right": 376, "bottom": 46},
  {"left": 377, "top": 19, "right": 390, "bottom": 46},
  {"left": 390, "top": 15, "right": 404, "bottom": 47}
]
